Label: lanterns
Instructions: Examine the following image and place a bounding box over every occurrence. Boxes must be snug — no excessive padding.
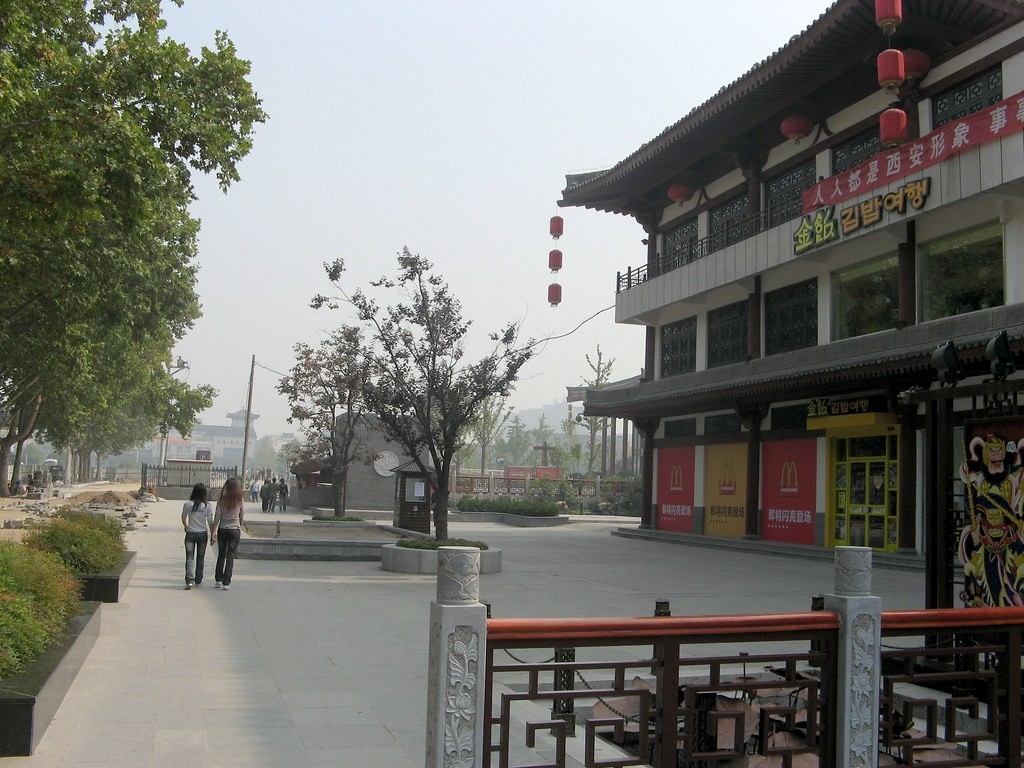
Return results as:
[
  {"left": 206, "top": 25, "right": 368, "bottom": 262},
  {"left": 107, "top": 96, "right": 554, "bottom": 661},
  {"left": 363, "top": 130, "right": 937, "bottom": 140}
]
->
[
  {"left": 780, "top": 112, "right": 814, "bottom": 146},
  {"left": 880, "top": 108, "right": 907, "bottom": 151},
  {"left": 548, "top": 249, "right": 563, "bottom": 274},
  {"left": 876, "top": 49, "right": 905, "bottom": 96},
  {"left": 875, "top": 0, "right": 904, "bottom": 34},
  {"left": 667, "top": 182, "right": 697, "bottom": 208},
  {"left": 902, "top": 48, "right": 931, "bottom": 87},
  {"left": 549, "top": 216, "right": 564, "bottom": 241},
  {"left": 548, "top": 283, "right": 563, "bottom": 308}
]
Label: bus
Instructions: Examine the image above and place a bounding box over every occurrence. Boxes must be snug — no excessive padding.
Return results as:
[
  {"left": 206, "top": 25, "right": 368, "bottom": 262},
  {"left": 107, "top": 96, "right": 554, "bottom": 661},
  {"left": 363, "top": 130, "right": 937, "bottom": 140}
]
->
[{"left": 45, "top": 459, "right": 57, "bottom": 469}]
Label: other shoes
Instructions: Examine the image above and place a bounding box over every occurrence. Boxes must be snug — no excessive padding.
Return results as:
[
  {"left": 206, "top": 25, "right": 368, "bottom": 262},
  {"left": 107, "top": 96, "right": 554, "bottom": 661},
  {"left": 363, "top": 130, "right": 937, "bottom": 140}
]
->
[
  {"left": 215, "top": 581, "right": 222, "bottom": 587},
  {"left": 222, "top": 584, "right": 231, "bottom": 589},
  {"left": 185, "top": 582, "right": 192, "bottom": 590},
  {"left": 195, "top": 578, "right": 203, "bottom": 587}
]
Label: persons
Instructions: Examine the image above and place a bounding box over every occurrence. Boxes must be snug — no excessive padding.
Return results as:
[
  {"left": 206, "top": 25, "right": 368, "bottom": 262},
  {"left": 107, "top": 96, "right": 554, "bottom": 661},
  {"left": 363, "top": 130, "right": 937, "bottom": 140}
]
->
[
  {"left": 211, "top": 478, "right": 243, "bottom": 591},
  {"left": 181, "top": 482, "right": 215, "bottom": 590},
  {"left": 246, "top": 467, "right": 288, "bottom": 513},
  {"left": 26, "top": 475, "right": 35, "bottom": 490},
  {"left": 14, "top": 481, "right": 24, "bottom": 495}
]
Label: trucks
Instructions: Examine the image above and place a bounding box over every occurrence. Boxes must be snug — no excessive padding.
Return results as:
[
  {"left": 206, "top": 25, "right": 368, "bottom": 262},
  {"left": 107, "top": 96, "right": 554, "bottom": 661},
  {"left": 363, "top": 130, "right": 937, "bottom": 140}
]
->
[{"left": 49, "top": 465, "right": 63, "bottom": 480}]
